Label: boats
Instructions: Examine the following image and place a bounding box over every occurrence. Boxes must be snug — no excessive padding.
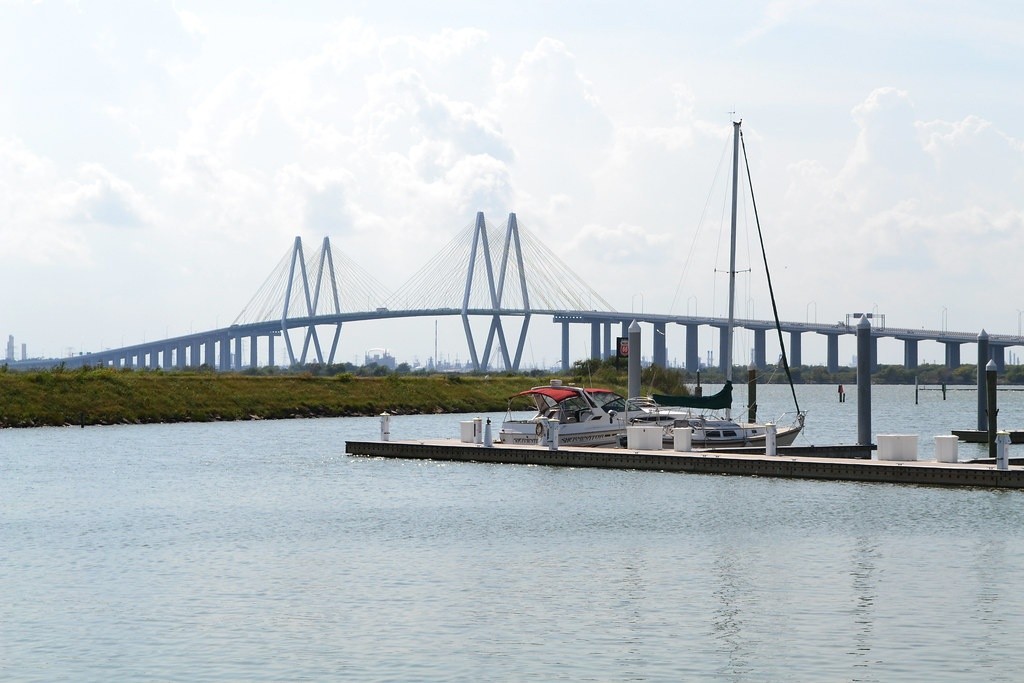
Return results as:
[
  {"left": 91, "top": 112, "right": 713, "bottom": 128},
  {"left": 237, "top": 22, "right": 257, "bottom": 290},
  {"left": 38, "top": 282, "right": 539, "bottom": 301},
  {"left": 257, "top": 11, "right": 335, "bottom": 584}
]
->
[{"left": 499, "top": 379, "right": 646, "bottom": 447}]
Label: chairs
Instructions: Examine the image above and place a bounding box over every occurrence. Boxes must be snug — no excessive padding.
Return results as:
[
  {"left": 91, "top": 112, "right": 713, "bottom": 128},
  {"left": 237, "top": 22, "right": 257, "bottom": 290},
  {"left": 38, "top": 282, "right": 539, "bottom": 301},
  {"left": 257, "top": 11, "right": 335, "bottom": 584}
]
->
[{"left": 567, "top": 417, "right": 576, "bottom": 423}]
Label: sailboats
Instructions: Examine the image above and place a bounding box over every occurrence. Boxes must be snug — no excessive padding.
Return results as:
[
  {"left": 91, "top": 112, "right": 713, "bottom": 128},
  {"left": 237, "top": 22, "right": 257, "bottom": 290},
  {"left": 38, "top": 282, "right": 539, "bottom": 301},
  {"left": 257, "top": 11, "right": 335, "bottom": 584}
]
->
[{"left": 613, "top": 112, "right": 809, "bottom": 451}]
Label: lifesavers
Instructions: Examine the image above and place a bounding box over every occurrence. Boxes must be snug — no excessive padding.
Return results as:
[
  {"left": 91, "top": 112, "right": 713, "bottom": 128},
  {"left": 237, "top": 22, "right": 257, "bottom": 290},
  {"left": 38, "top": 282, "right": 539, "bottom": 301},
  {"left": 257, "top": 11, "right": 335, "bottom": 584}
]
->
[{"left": 536, "top": 422, "right": 543, "bottom": 434}]
